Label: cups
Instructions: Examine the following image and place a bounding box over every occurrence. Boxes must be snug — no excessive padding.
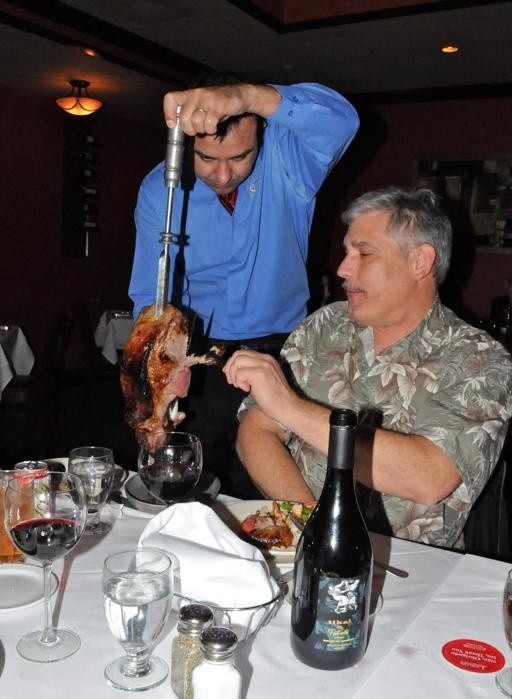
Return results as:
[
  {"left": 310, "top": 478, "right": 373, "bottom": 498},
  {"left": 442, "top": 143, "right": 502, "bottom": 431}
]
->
[
  {"left": 0, "top": 471, "right": 35, "bottom": 565},
  {"left": 139, "top": 433, "right": 205, "bottom": 505}
]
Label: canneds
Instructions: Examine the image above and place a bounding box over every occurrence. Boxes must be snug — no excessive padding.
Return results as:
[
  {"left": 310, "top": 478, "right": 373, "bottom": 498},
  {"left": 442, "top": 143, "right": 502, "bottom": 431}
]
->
[{"left": 11, "top": 460, "right": 52, "bottom": 516}]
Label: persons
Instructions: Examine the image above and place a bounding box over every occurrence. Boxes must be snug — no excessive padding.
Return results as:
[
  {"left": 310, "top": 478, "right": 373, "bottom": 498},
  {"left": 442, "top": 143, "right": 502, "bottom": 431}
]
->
[
  {"left": 124, "top": 77, "right": 362, "bottom": 501},
  {"left": 223, "top": 179, "right": 512, "bottom": 555}
]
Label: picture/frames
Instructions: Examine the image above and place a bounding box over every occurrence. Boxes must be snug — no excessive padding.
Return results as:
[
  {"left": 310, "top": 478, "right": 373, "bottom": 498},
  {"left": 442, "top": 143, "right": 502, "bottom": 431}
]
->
[{"left": 411, "top": 153, "right": 512, "bottom": 254}]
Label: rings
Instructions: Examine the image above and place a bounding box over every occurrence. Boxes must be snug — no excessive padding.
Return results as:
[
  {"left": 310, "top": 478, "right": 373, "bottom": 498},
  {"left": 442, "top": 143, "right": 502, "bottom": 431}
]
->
[{"left": 195, "top": 106, "right": 207, "bottom": 114}]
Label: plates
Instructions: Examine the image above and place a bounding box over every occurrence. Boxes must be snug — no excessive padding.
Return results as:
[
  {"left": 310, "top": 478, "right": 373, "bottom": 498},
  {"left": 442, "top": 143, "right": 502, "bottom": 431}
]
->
[
  {"left": 214, "top": 499, "right": 302, "bottom": 564},
  {"left": 125, "top": 470, "right": 222, "bottom": 515},
  {"left": 0, "top": 563, "right": 59, "bottom": 616}
]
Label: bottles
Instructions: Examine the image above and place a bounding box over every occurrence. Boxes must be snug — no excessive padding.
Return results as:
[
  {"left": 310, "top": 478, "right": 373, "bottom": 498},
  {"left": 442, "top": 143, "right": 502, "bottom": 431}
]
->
[
  {"left": 170, "top": 603, "right": 243, "bottom": 699},
  {"left": 290, "top": 410, "right": 375, "bottom": 672}
]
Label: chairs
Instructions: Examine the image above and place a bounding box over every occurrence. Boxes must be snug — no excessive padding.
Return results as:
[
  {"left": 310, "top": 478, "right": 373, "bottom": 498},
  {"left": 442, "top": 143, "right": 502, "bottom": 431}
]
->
[{"left": 0, "top": 300, "right": 122, "bottom": 466}]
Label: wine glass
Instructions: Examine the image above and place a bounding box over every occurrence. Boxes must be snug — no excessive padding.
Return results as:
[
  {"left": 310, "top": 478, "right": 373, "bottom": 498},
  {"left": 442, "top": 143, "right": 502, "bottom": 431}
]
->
[
  {"left": 493, "top": 566, "right": 512, "bottom": 699},
  {"left": 100, "top": 547, "right": 175, "bottom": 692},
  {"left": 5, "top": 471, "right": 89, "bottom": 663},
  {"left": 69, "top": 447, "right": 114, "bottom": 537}
]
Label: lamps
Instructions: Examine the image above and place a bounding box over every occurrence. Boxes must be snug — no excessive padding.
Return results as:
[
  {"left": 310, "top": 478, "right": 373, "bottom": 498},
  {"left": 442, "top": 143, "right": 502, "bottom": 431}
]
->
[{"left": 54, "top": 79, "right": 103, "bottom": 117}]
